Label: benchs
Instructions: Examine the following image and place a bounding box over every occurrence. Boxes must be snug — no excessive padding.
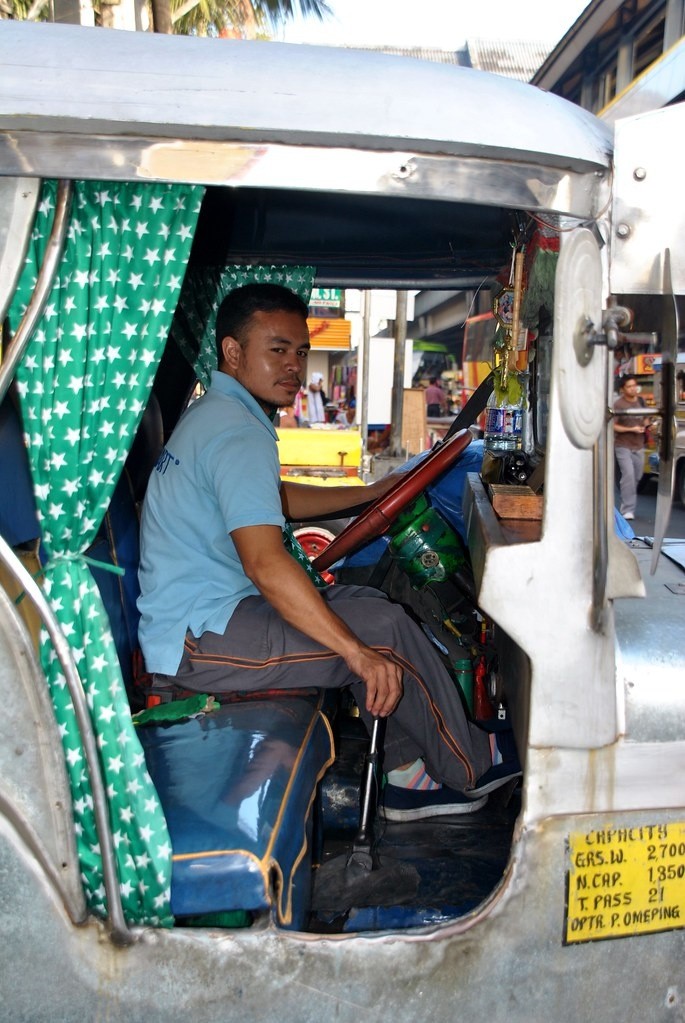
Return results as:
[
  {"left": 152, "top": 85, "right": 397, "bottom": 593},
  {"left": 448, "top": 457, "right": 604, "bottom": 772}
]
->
[{"left": 36, "top": 501, "right": 334, "bottom": 930}]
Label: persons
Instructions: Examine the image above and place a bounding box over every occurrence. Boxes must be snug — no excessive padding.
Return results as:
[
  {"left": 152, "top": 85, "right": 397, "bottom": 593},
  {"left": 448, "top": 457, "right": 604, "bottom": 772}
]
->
[
  {"left": 136, "top": 280, "right": 526, "bottom": 822},
  {"left": 676, "top": 369, "right": 685, "bottom": 402},
  {"left": 305, "top": 372, "right": 334, "bottom": 420},
  {"left": 610, "top": 373, "right": 656, "bottom": 521},
  {"left": 426, "top": 377, "right": 448, "bottom": 416}
]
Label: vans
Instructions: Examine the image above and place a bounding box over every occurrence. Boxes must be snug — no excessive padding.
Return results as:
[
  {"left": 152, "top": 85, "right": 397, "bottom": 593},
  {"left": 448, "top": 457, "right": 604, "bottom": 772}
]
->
[{"left": 1, "top": 18, "right": 685, "bottom": 1023}]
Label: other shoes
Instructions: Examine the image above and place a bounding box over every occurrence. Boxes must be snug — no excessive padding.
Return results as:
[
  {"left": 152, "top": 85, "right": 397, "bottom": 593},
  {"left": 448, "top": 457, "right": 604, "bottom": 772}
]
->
[
  {"left": 622, "top": 512, "right": 634, "bottom": 519},
  {"left": 379, "top": 782, "right": 488, "bottom": 822},
  {"left": 464, "top": 739, "right": 522, "bottom": 798}
]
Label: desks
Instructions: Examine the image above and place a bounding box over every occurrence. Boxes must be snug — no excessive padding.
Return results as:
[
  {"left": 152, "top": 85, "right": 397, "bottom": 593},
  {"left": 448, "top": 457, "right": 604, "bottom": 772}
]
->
[{"left": 275, "top": 428, "right": 362, "bottom": 466}]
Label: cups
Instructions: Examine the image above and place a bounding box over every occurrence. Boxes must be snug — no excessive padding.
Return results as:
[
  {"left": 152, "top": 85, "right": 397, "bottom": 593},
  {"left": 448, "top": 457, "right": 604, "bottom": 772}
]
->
[{"left": 455, "top": 659, "right": 472, "bottom": 721}]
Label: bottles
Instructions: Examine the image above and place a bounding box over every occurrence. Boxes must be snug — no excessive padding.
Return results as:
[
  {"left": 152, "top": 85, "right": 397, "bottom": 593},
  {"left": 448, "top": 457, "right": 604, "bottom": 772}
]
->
[{"left": 484, "top": 372, "right": 526, "bottom": 451}]
